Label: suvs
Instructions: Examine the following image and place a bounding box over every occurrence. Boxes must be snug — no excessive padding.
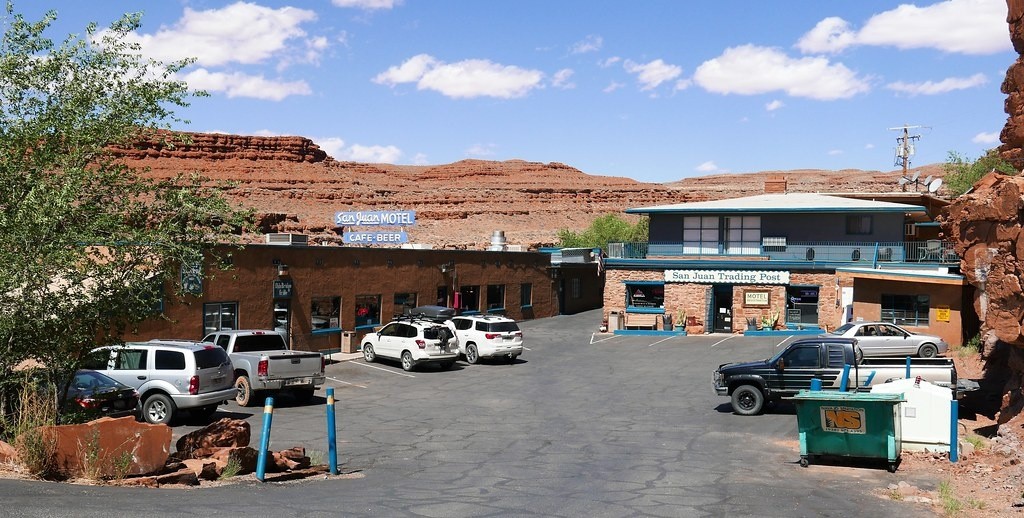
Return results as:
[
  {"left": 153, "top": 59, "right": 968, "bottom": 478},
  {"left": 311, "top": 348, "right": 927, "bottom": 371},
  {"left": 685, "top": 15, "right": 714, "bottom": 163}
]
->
[
  {"left": 70, "top": 339, "right": 239, "bottom": 426},
  {"left": 360, "top": 305, "right": 460, "bottom": 372},
  {"left": 449, "top": 314, "right": 525, "bottom": 365}
]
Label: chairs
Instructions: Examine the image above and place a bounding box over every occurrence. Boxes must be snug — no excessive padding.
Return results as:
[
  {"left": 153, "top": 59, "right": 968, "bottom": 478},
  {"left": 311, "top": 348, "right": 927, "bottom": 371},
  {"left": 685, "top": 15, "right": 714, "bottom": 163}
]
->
[{"left": 917, "top": 240, "right": 942, "bottom": 263}]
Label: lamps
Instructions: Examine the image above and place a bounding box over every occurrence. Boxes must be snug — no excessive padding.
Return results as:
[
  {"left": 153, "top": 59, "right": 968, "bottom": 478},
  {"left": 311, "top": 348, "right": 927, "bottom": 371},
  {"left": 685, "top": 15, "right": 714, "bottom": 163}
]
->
[
  {"left": 277, "top": 264, "right": 288, "bottom": 277},
  {"left": 442, "top": 264, "right": 449, "bottom": 274}
]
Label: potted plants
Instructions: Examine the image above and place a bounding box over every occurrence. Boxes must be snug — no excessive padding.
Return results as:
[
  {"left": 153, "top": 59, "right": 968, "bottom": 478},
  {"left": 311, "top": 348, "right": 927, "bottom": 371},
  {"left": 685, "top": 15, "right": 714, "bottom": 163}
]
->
[
  {"left": 762, "top": 304, "right": 781, "bottom": 330},
  {"left": 675, "top": 307, "right": 687, "bottom": 331},
  {"left": 745, "top": 316, "right": 757, "bottom": 330},
  {"left": 869, "top": 327, "right": 876, "bottom": 336}
]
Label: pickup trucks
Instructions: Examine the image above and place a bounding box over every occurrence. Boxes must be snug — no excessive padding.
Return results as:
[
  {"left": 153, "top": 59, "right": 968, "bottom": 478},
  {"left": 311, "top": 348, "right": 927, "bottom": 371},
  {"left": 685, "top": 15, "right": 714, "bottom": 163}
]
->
[
  {"left": 712, "top": 337, "right": 958, "bottom": 417},
  {"left": 200, "top": 328, "right": 326, "bottom": 407}
]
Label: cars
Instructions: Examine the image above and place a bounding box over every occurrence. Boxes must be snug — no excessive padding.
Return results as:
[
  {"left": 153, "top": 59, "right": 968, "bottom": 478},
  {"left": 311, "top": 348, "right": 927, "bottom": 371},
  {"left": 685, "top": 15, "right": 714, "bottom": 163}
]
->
[
  {"left": 0, "top": 367, "right": 143, "bottom": 440},
  {"left": 816, "top": 320, "right": 948, "bottom": 366}
]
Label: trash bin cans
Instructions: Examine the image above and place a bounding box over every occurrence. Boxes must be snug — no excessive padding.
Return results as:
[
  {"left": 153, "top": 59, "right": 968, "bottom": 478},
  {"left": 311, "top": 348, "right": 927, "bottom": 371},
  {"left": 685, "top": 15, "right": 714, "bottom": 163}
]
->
[
  {"left": 783, "top": 390, "right": 907, "bottom": 472},
  {"left": 608, "top": 310, "right": 623, "bottom": 333}
]
